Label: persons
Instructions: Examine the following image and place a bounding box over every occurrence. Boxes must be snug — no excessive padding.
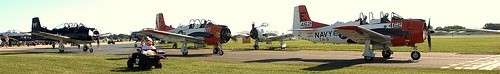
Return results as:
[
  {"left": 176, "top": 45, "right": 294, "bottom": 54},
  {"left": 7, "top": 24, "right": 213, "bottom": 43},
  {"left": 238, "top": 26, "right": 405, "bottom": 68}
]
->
[
  {"left": 97, "top": 37, "right": 100, "bottom": 48},
  {"left": 381, "top": 13, "right": 390, "bottom": 23},
  {"left": 360, "top": 15, "right": 369, "bottom": 25},
  {"left": 140, "top": 35, "right": 152, "bottom": 46}
]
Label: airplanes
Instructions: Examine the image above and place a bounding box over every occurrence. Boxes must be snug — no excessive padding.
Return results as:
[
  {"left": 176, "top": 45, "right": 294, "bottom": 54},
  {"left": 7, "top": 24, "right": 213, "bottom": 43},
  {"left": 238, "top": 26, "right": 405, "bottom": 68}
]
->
[
  {"left": 250, "top": 19, "right": 294, "bottom": 50},
  {"left": 291, "top": 4, "right": 435, "bottom": 61},
  {"left": 131, "top": 12, "right": 232, "bottom": 56},
  {"left": 5, "top": 16, "right": 99, "bottom": 53}
]
